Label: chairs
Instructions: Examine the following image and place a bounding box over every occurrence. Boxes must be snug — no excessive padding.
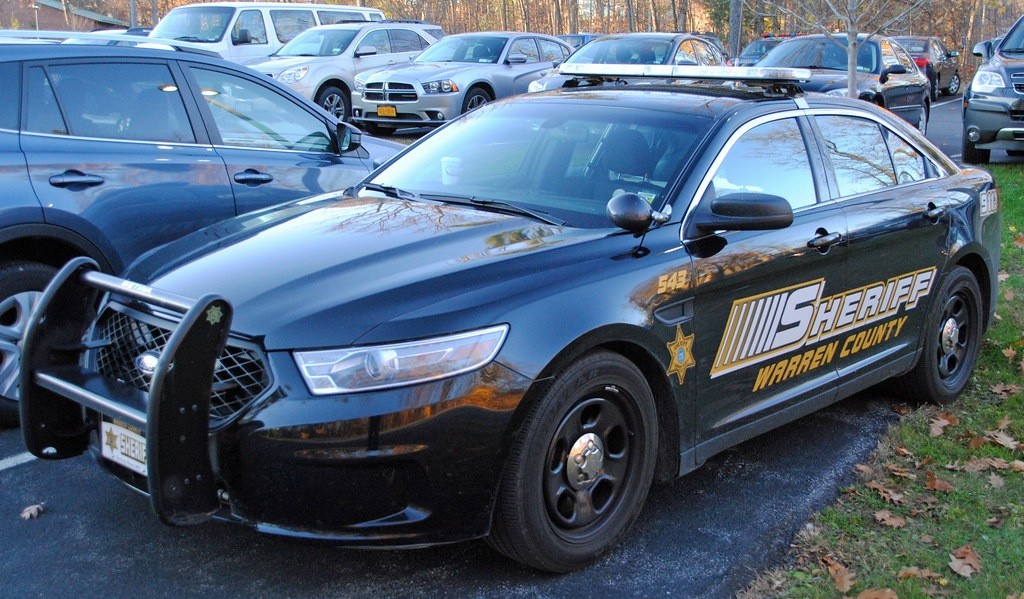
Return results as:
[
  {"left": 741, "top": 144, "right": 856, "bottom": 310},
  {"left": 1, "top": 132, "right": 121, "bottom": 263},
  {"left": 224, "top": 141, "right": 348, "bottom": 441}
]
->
[
  {"left": 48, "top": 78, "right": 84, "bottom": 134},
  {"left": 639, "top": 50, "right": 656, "bottom": 63},
  {"left": 615, "top": 46, "right": 633, "bottom": 63},
  {"left": 127, "top": 88, "right": 171, "bottom": 139},
  {"left": 472, "top": 46, "right": 490, "bottom": 60},
  {"left": 593, "top": 128, "right": 651, "bottom": 198}
]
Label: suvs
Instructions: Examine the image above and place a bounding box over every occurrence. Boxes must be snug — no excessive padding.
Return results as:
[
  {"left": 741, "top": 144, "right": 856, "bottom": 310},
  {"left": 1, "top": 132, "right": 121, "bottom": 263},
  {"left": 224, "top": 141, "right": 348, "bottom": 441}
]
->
[{"left": 242, "top": 19, "right": 458, "bottom": 124}]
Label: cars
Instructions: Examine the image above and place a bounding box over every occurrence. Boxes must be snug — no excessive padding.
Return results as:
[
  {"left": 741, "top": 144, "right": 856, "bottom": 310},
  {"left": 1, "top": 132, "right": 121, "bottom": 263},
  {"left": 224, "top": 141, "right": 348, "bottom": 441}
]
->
[
  {"left": 527, "top": 31, "right": 736, "bottom": 100},
  {"left": 727, "top": 34, "right": 792, "bottom": 67},
  {"left": 887, "top": 35, "right": 961, "bottom": 101},
  {"left": 1, "top": 29, "right": 410, "bottom": 428},
  {"left": 18, "top": 59, "right": 1001, "bottom": 576},
  {"left": 721, "top": 32, "right": 932, "bottom": 138},
  {"left": 351, "top": 29, "right": 595, "bottom": 137},
  {"left": 961, "top": 13, "right": 1024, "bottom": 165}
]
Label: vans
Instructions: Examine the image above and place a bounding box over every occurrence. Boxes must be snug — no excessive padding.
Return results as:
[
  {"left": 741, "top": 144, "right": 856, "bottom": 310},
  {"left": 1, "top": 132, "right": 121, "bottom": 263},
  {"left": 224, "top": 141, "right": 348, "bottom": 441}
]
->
[{"left": 132, "top": 1, "right": 397, "bottom": 68}]
